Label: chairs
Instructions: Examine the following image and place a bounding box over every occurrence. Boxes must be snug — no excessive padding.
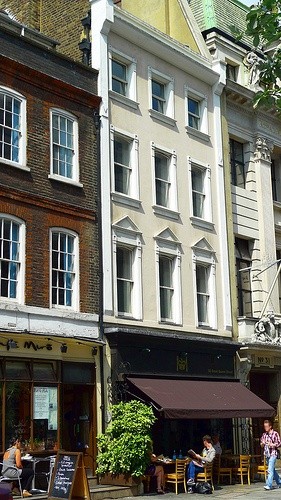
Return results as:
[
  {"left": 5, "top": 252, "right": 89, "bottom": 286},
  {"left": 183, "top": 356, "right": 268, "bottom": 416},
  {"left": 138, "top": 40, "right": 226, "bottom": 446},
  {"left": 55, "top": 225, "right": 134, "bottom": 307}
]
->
[
  {"left": 147, "top": 454, "right": 268, "bottom": 495},
  {"left": 0, "top": 463, "right": 23, "bottom": 498}
]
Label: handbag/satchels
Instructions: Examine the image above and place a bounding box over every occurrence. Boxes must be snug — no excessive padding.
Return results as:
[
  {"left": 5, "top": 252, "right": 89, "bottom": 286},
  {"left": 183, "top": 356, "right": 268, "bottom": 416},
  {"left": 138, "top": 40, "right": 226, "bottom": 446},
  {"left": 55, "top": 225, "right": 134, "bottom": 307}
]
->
[
  {"left": 193, "top": 481, "right": 213, "bottom": 494},
  {"left": 276, "top": 449, "right": 280, "bottom": 459}
]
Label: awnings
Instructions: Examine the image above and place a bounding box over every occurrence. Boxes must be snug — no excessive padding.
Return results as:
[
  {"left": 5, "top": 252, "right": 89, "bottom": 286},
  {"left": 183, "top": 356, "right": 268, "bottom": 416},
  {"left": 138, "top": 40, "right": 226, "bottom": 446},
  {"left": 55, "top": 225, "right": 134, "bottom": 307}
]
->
[{"left": 124, "top": 376, "right": 275, "bottom": 420}]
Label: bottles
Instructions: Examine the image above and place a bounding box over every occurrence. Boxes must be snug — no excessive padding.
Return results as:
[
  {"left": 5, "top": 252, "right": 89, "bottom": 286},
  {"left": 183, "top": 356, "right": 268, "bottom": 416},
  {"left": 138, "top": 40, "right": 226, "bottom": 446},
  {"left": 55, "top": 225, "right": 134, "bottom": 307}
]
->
[
  {"left": 178, "top": 450, "right": 182, "bottom": 459},
  {"left": 173, "top": 450, "right": 176, "bottom": 460}
]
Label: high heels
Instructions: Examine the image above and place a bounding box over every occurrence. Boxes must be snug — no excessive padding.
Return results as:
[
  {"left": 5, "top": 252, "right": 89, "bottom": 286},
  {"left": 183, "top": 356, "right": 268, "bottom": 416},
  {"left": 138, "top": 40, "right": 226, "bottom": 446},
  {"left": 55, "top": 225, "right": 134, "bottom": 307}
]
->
[
  {"left": 12, "top": 489, "right": 20, "bottom": 496},
  {"left": 23, "top": 490, "right": 32, "bottom": 498}
]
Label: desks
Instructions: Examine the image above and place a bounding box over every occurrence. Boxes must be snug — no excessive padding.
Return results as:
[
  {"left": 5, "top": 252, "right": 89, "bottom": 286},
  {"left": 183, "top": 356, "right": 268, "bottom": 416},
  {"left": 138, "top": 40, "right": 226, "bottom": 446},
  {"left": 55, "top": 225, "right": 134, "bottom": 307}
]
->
[
  {"left": 224, "top": 455, "right": 262, "bottom": 484},
  {"left": 156, "top": 459, "right": 176, "bottom": 493},
  {"left": 21, "top": 456, "right": 54, "bottom": 494}
]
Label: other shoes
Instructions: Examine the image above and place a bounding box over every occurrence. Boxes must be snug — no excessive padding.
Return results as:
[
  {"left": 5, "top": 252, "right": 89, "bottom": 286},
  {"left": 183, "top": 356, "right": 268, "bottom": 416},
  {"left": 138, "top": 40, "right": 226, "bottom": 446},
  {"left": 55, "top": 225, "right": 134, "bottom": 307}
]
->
[
  {"left": 264, "top": 485, "right": 271, "bottom": 490},
  {"left": 156, "top": 488, "right": 165, "bottom": 494},
  {"left": 187, "top": 486, "right": 193, "bottom": 494},
  {"left": 187, "top": 478, "right": 196, "bottom": 486},
  {"left": 273, "top": 483, "right": 281, "bottom": 489}
]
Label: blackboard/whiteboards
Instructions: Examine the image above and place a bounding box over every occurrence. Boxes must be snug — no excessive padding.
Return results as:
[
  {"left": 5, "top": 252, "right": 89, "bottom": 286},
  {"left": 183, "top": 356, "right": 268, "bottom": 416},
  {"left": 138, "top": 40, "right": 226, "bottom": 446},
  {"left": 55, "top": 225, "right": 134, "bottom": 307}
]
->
[{"left": 48, "top": 452, "right": 90, "bottom": 500}]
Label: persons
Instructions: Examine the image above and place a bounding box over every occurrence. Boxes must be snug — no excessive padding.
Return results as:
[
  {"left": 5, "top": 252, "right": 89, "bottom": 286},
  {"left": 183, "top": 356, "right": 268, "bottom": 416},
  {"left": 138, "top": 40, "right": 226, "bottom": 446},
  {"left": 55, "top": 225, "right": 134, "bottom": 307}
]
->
[
  {"left": 1, "top": 436, "right": 35, "bottom": 497},
  {"left": 146, "top": 453, "right": 164, "bottom": 494},
  {"left": 260, "top": 419, "right": 281, "bottom": 490},
  {"left": 186, "top": 432, "right": 222, "bottom": 494}
]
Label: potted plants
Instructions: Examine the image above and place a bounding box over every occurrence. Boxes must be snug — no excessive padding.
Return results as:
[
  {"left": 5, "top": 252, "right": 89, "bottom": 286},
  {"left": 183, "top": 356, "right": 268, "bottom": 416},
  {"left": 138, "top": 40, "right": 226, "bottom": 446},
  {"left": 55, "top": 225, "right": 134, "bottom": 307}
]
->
[{"left": 94, "top": 400, "right": 158, "bottom": 487}]
